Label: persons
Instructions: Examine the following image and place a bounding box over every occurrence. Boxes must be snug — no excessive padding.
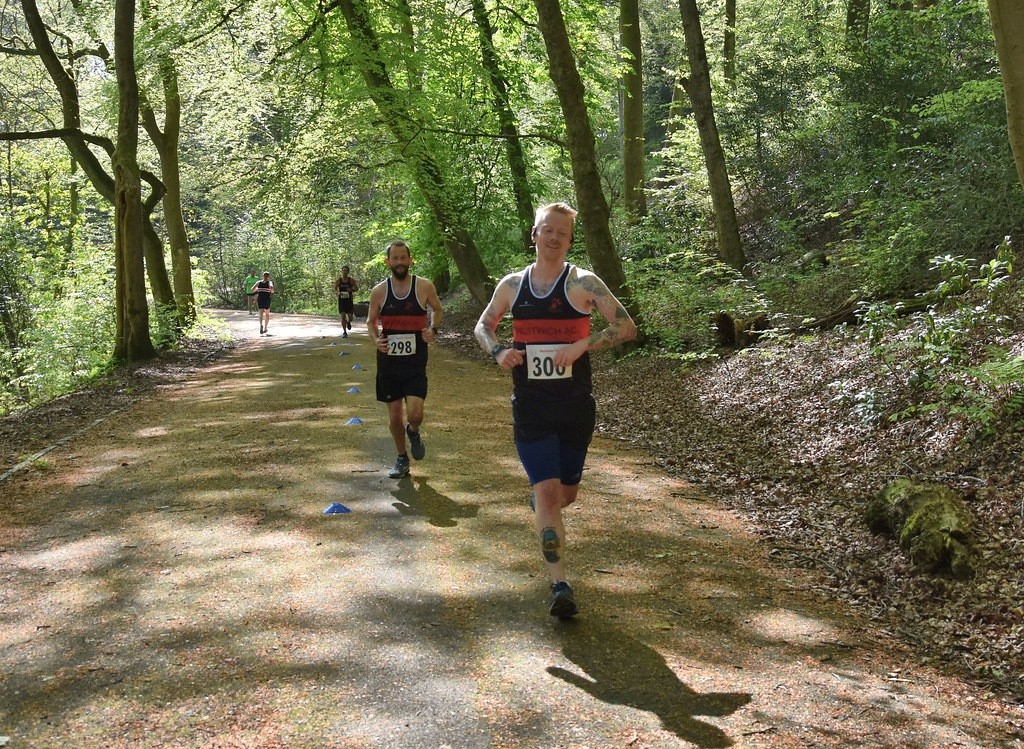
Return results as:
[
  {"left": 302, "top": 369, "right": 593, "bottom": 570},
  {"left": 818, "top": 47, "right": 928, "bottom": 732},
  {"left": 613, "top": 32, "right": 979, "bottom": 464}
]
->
[
  {"left": 476, "top": 203, "right": 636, "bottom": 618},
  {"left": 251, "top": 272, "right": 274, "bottom": 335},
  {"left": 334, "top": 266, "right": 358, "bottom": 339},
  {"left": 366, "top": 242, "right": 443, "bottom": 479},
  {"left": 243, "top": 269, "right": 259, "bottom": 315}
]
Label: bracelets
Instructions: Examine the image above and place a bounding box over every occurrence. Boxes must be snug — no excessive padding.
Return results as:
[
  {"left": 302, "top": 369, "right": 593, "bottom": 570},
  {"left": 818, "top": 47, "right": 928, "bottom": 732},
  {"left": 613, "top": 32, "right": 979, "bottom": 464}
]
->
[{"left": 429, "top": 324, "right": 439, "bottom": 337}]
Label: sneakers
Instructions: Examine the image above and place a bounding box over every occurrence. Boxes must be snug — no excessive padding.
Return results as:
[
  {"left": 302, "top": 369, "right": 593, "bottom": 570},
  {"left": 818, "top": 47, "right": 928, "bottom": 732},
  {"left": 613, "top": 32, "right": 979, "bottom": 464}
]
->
[
  {"left": 549, "top": 579, "right": 578, "bottom": 617},
  {"left": 406, "top": 423, "right": 425, "bottom": 460},
  {"left": 530, "top": 491, "right": 536, "bottom": 511},
  {"left": 388, "top": 457, "right": 410, "bottom": 477}
]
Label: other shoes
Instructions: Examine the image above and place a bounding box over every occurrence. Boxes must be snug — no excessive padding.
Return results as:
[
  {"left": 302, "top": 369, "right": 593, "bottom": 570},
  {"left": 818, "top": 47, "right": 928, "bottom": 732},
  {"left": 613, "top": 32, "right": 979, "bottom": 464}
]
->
[
  {"left": 343, "top": 333, "right": 347, "bottom": 338},
  {"left": 260, "top": 325, "right": 263, "bottom": 334},
  {"left": 264, "top": 329, "right": 268, "bottom": 332},
  {"left": 347, "top": 322, "right": 351, "bottom": 330}
]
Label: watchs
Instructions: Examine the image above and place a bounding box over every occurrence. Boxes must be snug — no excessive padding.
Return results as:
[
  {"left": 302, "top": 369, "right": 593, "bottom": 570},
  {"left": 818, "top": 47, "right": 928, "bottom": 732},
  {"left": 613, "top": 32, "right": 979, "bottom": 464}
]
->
[{"left": 489, "top": 343, "right": 504, "bottom": 363}]
